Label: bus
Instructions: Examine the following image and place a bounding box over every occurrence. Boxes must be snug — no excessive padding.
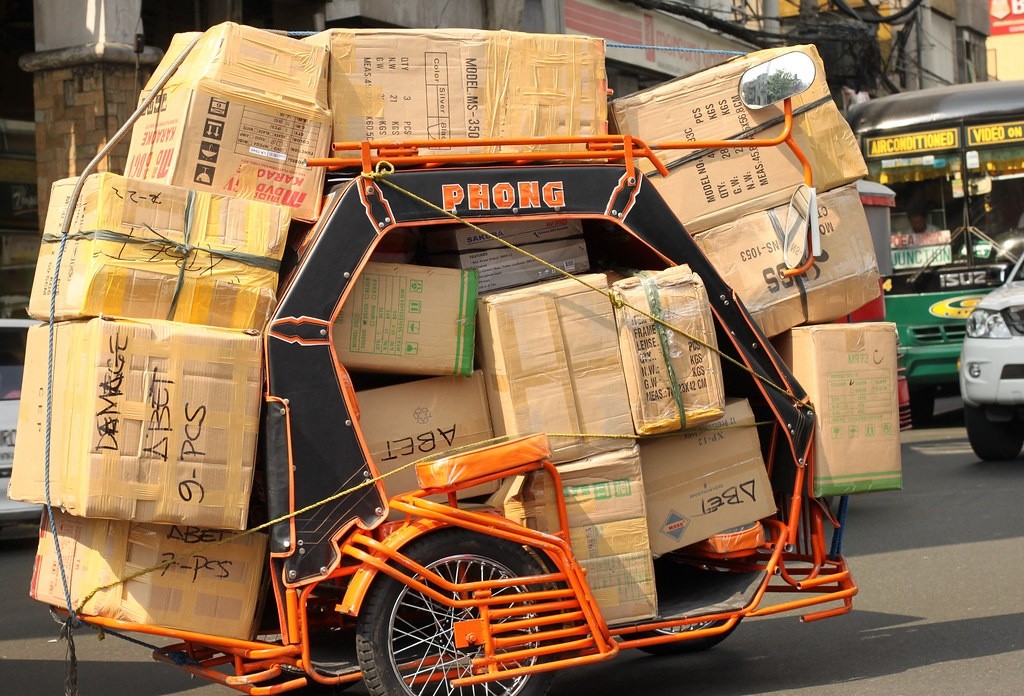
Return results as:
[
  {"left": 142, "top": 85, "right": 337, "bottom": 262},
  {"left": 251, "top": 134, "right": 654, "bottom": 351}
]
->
[{"left": 843, "top": 80, "right": 1024, "bottom": 432}]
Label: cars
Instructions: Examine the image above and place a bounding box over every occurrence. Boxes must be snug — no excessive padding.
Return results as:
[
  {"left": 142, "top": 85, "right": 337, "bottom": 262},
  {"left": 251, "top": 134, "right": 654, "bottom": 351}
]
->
[
  {"left": 0, "top": 317, "right": 52, "bottom": 530},
  {"left": 957, "top": 248, "right": 1024, "bottom": 464}
]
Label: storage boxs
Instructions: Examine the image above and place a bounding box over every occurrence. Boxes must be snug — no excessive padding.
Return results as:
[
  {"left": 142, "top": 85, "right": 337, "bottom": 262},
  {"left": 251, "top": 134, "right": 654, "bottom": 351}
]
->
[{"left": 10, "top": 22, "right": 905, "bottom": 641}]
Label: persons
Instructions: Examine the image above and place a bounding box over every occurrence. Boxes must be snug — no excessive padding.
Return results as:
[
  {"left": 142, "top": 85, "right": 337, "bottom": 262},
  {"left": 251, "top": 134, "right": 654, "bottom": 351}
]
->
[
  {"left": 970, "top": 181, "right": 1020, "bottom": 241},
  {"left": 903, "top": 204, "right": 943, "bottom": 233}
]
